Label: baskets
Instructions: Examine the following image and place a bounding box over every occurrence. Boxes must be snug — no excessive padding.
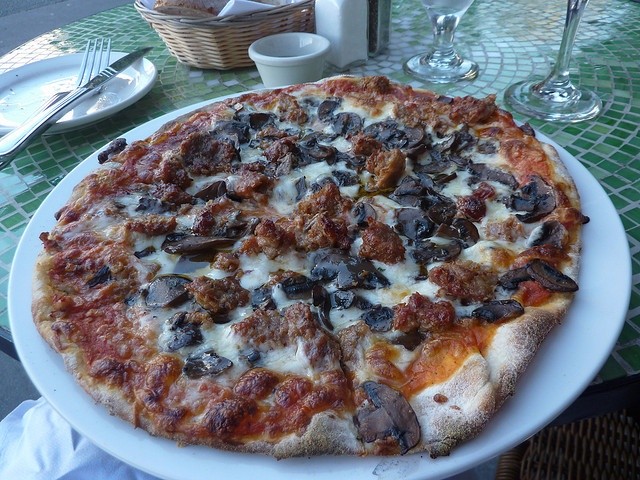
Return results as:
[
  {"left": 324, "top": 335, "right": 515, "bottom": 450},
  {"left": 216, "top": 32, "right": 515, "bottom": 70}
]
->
[{"left": 133, "top": 0, "right": 315, "bottom": 70}]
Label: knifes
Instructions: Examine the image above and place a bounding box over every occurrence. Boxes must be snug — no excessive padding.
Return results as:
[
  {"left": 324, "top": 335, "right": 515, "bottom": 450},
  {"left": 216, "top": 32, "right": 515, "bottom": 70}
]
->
[{"left": 1, "top": 46, "right": 155, "bottom": 154}]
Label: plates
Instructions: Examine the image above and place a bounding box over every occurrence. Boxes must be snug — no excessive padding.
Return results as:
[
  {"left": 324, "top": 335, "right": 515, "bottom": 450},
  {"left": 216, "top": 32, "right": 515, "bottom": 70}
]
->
[
  {"left": 2, "top": 52, "right": 156, "bottom": 134},
  {"left": 6, "top": 82, "right": 632, "bottom": 480}
]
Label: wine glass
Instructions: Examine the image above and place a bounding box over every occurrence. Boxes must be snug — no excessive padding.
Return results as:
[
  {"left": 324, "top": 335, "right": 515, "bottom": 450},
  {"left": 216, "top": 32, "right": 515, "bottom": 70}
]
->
[
  {"left": 505, "top": 2, "right": 603, "bottom": 119},
  {"left": 404, "top": 1, "right": 480, "bottom": 82}
]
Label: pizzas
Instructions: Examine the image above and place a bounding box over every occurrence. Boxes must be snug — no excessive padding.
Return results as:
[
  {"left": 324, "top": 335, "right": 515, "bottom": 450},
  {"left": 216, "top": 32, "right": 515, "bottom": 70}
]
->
[{"left": 31, "top": 75, "right": 589, "bottom": 461}]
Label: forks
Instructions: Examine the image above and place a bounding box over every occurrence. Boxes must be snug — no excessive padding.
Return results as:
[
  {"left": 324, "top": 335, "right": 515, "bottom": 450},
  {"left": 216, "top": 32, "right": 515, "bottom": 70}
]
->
[{"left": 25, "top": 38, "right": 113, "bottom": 120}]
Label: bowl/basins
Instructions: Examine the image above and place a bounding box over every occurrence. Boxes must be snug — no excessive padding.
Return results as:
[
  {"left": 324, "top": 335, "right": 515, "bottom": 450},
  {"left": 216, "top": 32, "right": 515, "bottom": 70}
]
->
[{"left": 249, "top": 32, "right": 330, "bottom": 87}]
